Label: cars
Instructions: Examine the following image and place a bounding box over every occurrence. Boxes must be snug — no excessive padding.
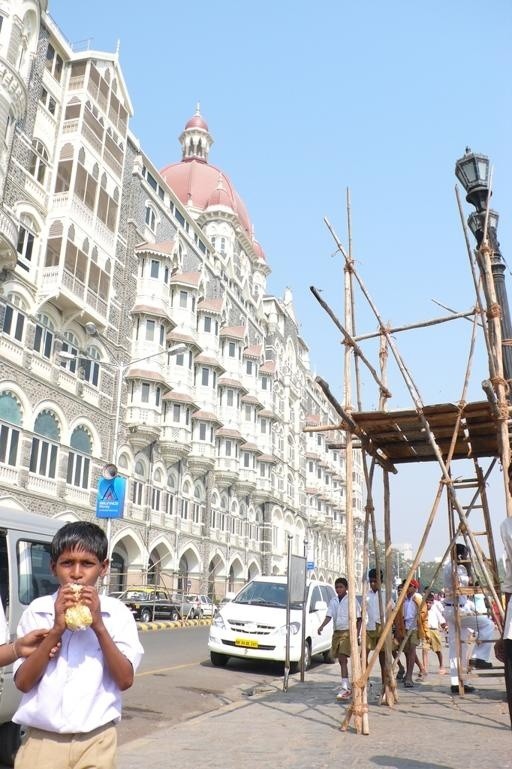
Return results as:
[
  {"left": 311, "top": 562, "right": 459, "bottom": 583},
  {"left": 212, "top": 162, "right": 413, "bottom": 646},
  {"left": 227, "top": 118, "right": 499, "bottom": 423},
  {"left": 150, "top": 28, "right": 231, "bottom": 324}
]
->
[{"left": 105, "top": 590, "right": 217, "bottom": 621}]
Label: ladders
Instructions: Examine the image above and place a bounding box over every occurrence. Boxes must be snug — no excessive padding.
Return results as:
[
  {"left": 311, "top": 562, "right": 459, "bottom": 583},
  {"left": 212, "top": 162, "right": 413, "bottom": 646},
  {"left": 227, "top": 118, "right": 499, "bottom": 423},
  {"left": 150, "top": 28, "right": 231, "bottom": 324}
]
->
[{"left": 447, "top": 467, "right": 508, "bottom": 699}]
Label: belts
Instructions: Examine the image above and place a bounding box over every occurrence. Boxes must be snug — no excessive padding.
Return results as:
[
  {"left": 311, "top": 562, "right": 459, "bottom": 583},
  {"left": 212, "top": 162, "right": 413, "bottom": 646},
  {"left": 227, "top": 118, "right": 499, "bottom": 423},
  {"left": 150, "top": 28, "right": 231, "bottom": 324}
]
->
[{"left": 445, "top": 603, "right": 463, "bottom": 608}]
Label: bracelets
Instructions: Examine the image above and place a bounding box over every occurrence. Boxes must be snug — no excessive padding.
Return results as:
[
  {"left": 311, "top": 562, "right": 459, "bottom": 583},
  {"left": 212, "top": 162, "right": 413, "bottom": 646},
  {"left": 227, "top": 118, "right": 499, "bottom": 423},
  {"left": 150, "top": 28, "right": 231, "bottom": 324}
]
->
[{"left": 13, "top": 641, "right": 19, "bottom": 659}]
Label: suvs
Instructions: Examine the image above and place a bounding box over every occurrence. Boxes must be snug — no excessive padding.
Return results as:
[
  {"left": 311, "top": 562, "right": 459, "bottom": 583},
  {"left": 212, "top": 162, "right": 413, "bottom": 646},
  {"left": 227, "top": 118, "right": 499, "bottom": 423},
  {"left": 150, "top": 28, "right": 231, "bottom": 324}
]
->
[{"left": 208, "top": 575, "right": 337, "bottom": 670}]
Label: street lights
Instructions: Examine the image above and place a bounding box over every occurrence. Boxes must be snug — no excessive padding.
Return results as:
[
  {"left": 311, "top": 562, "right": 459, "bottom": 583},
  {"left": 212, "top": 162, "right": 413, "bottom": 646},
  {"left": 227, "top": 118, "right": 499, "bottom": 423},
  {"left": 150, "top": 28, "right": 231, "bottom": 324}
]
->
[
  {"left": 454, "top": 145, "right": 511, "bottom": 498},
  {"left": 86, "top": 322, "right": 187, "bottom": 595}
]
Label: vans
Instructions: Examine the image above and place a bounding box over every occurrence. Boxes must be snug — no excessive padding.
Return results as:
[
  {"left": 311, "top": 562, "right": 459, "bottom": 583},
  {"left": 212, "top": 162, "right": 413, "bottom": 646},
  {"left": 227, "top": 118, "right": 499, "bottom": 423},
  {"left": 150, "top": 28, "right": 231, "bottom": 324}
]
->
[{"left": 2, "top": 505, "right": 66, "bottom": 723}]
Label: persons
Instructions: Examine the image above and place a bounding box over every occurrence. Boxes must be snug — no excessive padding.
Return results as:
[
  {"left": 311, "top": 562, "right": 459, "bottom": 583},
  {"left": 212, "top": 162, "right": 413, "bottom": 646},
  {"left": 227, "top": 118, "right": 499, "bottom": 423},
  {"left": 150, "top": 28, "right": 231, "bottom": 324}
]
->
[
  {"left": 494, "top": 460, "right": 512, "bottom": 733},
  {"left": 12, "top": 520, "right": 145, "bottom": 769},
  {"left": 318, "top": 544, "right": 507, "bottom": 700},
  {"left": 0, "top": 594, "right": 64, "bottom": 701}
]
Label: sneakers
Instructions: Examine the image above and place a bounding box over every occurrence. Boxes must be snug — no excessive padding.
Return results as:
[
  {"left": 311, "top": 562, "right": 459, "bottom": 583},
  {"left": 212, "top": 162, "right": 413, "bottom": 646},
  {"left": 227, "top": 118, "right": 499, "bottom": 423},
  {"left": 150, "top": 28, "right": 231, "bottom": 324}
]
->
[{"left": 336, "top": 659, "right": 492, "bottom": 702}]
